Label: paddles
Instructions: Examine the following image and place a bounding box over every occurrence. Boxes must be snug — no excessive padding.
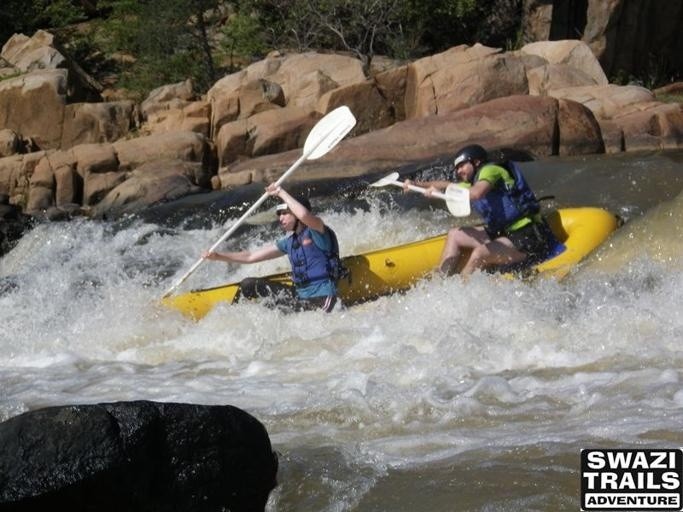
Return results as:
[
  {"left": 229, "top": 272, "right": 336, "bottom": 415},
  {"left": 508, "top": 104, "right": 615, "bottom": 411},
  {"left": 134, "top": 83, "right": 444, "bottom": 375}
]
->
[
  {"left": 369, "top": 172, "right": 470, "bottom": 218},
  {"left": 163, "top": 105, "right": 357, "bottom": 298}
]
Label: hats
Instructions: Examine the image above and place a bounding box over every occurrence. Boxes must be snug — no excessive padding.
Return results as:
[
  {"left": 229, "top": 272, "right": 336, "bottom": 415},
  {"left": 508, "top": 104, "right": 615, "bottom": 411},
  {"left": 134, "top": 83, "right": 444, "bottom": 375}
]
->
[{"left": 452, "top": 145, "right": 487, "bottom": 169}]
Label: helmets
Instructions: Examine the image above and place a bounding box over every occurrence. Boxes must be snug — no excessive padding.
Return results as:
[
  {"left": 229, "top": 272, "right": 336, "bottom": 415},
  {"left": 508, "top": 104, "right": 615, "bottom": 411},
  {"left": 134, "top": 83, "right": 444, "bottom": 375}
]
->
[{"left": 276, "top": 195, "right": 311, "bottom": 216}]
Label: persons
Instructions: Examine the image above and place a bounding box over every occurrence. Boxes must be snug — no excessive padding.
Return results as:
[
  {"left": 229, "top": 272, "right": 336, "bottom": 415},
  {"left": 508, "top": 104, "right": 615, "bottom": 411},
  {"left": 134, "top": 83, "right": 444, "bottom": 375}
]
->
[
  {"left": 202, "top": 182, "right": 342, "bottom": 315},
  {"left": 402, "top": 144, "right": 551, "bottom": 278}
]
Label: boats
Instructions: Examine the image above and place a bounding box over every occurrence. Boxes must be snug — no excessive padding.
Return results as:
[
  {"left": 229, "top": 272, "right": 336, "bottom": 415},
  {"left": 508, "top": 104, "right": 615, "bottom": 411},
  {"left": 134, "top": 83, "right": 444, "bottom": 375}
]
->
[{"left": 157, "top": 207, "right": 624, "bottom": 320}]
